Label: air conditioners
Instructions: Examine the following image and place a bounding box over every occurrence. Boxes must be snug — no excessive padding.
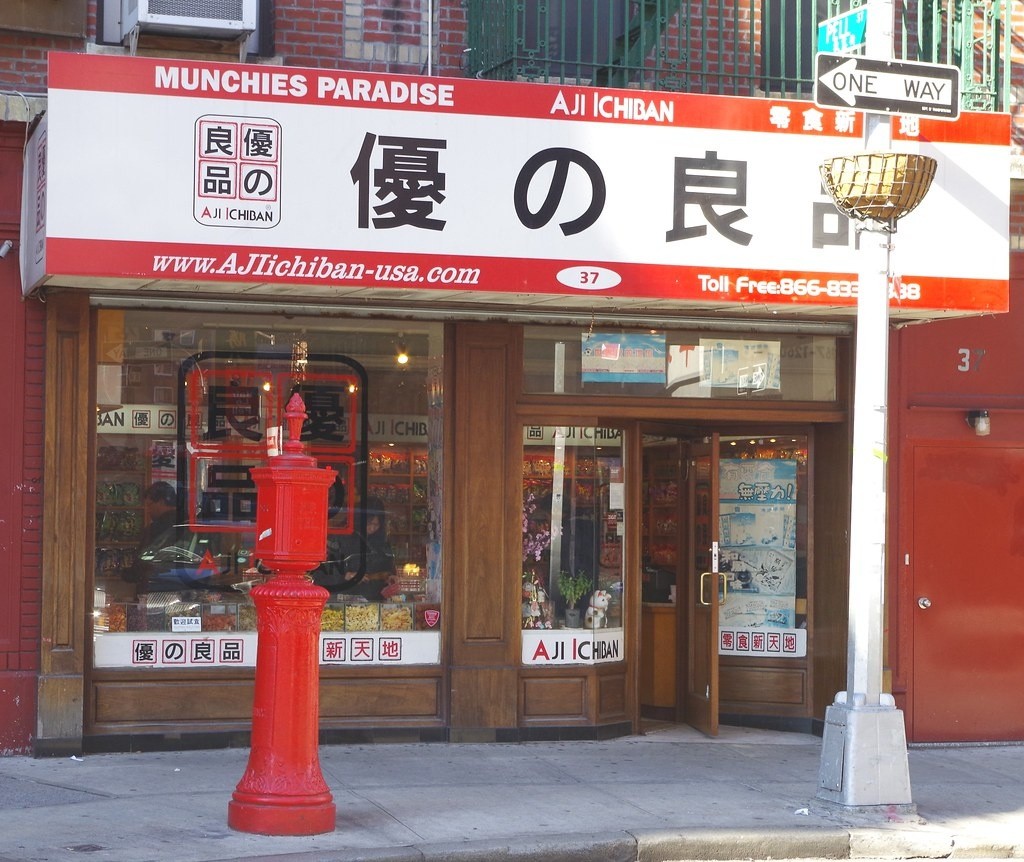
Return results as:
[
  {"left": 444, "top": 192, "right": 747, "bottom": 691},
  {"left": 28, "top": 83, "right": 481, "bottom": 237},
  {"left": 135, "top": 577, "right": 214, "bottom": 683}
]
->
[{"left": 118, "top": 1, "right": 257, "bottom": 61}]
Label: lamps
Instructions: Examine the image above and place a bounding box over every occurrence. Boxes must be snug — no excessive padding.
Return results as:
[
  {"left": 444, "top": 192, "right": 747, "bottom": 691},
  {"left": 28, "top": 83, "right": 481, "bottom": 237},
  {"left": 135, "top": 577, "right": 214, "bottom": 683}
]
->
[{"left": 965, "top": 411, "right": 993, "bottom": 435}]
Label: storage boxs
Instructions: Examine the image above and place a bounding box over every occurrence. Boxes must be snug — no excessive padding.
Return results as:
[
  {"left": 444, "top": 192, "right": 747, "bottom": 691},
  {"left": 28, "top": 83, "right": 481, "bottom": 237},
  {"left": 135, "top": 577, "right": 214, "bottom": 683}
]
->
[{"left": 95, "top": 597, "right": 441, "bottom": 632}]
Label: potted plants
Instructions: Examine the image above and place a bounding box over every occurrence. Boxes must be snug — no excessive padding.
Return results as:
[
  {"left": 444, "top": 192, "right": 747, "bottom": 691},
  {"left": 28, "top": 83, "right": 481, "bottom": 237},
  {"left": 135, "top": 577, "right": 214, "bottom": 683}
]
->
[{"left": 555, "top": 571, "right": 590, "bottom": 626}]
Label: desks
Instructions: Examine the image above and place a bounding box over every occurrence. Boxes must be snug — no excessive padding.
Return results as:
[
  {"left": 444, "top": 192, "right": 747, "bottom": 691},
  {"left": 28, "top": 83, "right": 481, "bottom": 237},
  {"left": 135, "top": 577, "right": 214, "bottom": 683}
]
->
[{"left": 638, "top": 601, "right": 708, "bottom": 708}]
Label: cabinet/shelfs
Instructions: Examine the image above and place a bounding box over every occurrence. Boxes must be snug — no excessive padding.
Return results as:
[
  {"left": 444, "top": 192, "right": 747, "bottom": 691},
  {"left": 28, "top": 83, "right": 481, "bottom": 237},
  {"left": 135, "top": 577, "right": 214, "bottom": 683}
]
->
[
  {"left": 690, "top": 447, "right": 812, "bottom": 580},
  {"left": 324, "top": 455, "right": 684, "bottom": 595},
  {"left": 91, "top": 443, "right": 153, "bottom": 581}
]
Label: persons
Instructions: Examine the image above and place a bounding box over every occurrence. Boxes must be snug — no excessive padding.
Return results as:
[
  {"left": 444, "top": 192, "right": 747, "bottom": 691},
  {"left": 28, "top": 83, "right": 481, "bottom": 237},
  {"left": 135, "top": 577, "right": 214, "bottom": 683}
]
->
[
  {"left": 327, "top": 495, "right": 398, "bottom": 602},
  {"left": 540, "top": 492, "right": 599, "bottom": 617},
  {"left": 119, "top": 481, "right": 211, "bottom": 603}
]
